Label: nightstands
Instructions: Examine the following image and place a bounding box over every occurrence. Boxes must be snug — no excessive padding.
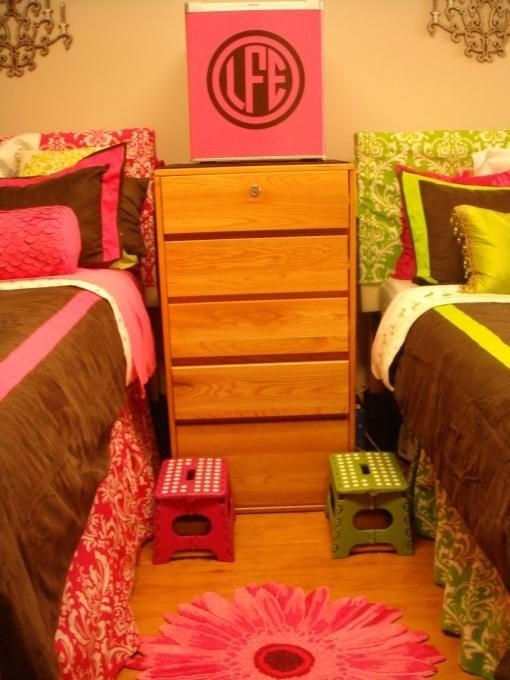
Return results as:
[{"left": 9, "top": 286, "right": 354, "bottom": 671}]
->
[{"left": 154, "top": 161, "right": 359, "bottom": 515}]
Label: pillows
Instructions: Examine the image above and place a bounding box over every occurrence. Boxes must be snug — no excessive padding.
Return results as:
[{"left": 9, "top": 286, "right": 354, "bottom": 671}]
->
[
  {"left": 388, "top": 158, "right": 510, "bottom": 296},
  {"left": 0, "top": 139, "right": 150, "bottom": 281}
]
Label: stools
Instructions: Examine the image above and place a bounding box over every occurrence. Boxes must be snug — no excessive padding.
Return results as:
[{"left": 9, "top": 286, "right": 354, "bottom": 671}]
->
[
  {"left": 153, "top": 454, "right": 239, "bottom": 565},
  {"left": 324, "top": 452, "right": 414, "bottom": 559}
]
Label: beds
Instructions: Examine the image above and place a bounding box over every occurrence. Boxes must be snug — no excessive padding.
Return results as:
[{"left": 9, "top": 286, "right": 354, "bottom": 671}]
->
[
  {"left": 353, "top": 128, "right": 510, "bottom": 679},
  {"left": 0, "top": 130, "right": 160, "bottom": 680}
]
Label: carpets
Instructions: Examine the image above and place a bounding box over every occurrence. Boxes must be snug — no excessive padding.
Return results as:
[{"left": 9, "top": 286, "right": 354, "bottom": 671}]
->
[{"left": 121, "top": 579, "right": 447, "bottom": 679}]
